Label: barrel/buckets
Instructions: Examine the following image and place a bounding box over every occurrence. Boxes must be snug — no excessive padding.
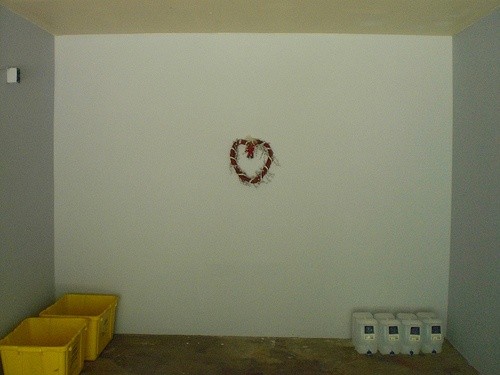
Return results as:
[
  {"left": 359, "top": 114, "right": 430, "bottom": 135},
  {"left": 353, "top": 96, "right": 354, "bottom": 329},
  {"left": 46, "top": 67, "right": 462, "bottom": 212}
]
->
[
  {"left": 397, "top": 312, "right": 423, "bottom": 356},
  {"left": 416, "top": 312, "right": 444, "bottom": 355},
  {"left": 352, "top": 312, "right": 378, "bottom": 356},
  {"left": 373, "top": 312, "right": 401, "bottom": 356}
]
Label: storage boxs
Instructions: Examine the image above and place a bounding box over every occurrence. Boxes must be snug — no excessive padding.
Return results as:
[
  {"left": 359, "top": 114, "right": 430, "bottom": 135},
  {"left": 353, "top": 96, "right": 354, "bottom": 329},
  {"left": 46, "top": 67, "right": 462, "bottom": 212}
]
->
[
  {"left": 39, "top": 292, "right": 119, "bottom": 362},
  {"left": 0, "top": 316, "right": 90, "bottom": 375}
]
